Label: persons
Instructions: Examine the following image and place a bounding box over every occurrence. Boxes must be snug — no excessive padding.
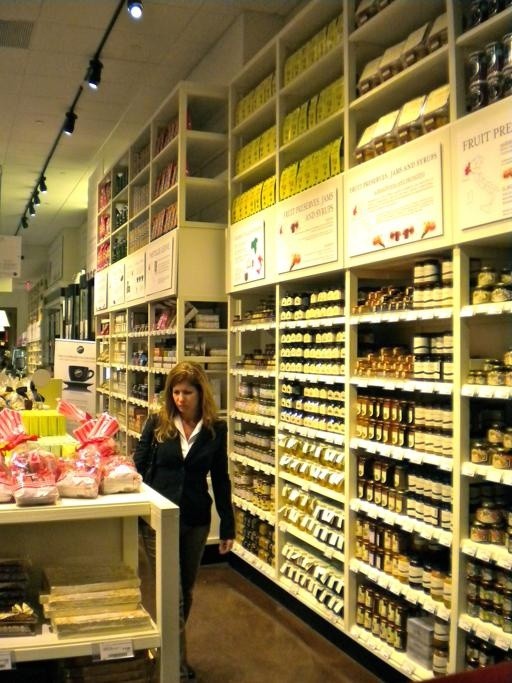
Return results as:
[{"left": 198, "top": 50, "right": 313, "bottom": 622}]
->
[{"left": 131, "top": 360, "right": 236, "bottom": 680}]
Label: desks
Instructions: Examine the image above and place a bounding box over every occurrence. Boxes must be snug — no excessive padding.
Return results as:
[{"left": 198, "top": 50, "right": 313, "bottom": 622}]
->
[{"left": 0, "top": 431, "right": 181, "bottom": 683}]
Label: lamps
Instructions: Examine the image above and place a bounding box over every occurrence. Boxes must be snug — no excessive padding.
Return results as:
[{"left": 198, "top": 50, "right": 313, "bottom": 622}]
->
[
  {"left": 0, "top": 309, "right": 12, "bottom": 354},
  {"left": 14, "top": 57, "right": 106, "bottom": 236}
]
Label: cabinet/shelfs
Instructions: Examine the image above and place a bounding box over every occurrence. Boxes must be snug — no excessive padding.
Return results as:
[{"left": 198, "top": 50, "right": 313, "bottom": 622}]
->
[
  {"left": 26, "top": 278, "right": 50, "bottom": 374},
  {"left": 93, "top": 0, "right": 511, "bottom": 683}
]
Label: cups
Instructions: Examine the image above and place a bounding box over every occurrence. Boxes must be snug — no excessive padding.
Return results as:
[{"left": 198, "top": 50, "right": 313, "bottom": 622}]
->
[{"left": 69, "top": 365, "right": 94, "bottom": 381}]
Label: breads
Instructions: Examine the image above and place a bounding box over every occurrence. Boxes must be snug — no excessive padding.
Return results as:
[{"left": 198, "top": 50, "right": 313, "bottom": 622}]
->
[{"left": 1, "top": 436, "right": 144, "bottom": 507}]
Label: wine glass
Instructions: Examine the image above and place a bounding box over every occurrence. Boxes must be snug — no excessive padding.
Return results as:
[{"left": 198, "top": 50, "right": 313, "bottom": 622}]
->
[{"left": 12, "top": 350, "right": 26, "bottom": 381}]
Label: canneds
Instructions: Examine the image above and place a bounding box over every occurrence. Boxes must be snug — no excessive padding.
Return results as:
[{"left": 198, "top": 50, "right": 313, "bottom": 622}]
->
[
  {"left": 461, "top": 0, "right": 512, "bottom": 114},
  {"left": 352, "top": 255, "right": 512, "bottom": 678},
  {"left": 233, "top": 380, "right": 276, "bottom": 515}
]
